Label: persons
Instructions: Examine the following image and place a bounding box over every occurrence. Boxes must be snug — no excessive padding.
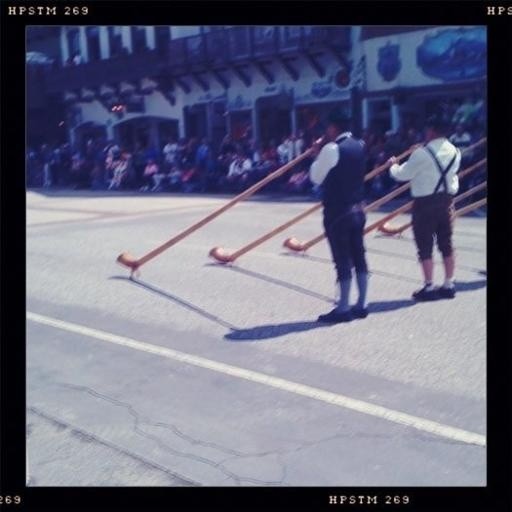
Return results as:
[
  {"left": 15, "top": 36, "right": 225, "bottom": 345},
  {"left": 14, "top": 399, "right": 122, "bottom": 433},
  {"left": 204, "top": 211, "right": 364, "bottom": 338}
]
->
[
  {"left": 310, "top": 109, "right": 370, "bottom": 323},
  {"left": 387, "top": 114, "right": 462, "bottom": 301},
  {"left": 25, "top": 78, "right": 487, "bottom": 215},
  {"left": 65, "top": 49, "right": 85, "bottom": 71}
]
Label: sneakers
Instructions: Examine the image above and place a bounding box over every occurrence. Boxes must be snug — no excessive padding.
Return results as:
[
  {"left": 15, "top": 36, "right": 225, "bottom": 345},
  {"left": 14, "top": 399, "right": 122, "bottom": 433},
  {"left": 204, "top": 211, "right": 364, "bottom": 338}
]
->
[
  {"left": 440, "top": 288, "right": 456, "bottom": 298},
  {"left": 319, "top": 311, "right": 354, "bottom": 322},
  {"left": 348, "top": 306, "right": 368, "bottom": 318},
  {"left": 414, "top": 288, "right": 440, "bottom": 299}
]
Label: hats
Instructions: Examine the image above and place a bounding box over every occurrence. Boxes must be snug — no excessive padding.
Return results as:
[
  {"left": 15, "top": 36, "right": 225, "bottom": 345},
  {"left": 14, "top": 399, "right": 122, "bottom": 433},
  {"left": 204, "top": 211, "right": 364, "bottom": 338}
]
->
[
  {"left": 321, "top": 105, "right": 353, "bottom": 123},
  {"left": 420, "top": 111, "right": 451, "bottom": 127}
]
